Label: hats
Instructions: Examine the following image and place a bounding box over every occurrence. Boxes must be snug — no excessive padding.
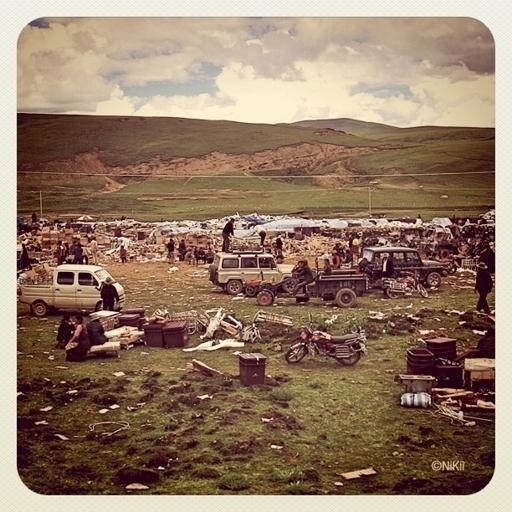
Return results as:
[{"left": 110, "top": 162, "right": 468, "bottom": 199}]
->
[
  {"left": 89, "top": 314, "right": 100, "bottom": 323},
  {"left": 104, "top": 276, "right": 113, "bottom": 284}
]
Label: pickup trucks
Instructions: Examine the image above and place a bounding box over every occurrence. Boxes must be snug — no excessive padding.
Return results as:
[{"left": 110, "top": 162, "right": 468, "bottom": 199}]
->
[{"left": 20, "top": 263, "right": 126, "bottom": 318}]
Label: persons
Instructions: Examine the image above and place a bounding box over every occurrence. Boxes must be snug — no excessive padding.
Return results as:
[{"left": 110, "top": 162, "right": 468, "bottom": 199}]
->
[
  {"left": 55, "top": 313, "right": 76, "bottom": 349},
  {"left": 473, "top": 261, "right": 493, "bottom": 314},
  {"left": 100, "top": 276, "right": 119, "bottom": 310},
  {"left": 17, "top": 211, "right": 494, "bottom": 296},
  {"left": 85, "top": 313, "right": 107, "bottom": 358},
  {"left": 65, "top": 315, "right": 90, "bottom": 362}
]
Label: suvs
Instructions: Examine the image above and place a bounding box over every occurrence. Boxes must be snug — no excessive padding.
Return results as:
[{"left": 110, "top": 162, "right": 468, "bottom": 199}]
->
[
  {"left": 360, "top": 246, "right": 448, "bottom": 289},
  {"left": 208, "top": 248, "right": 298, "bottom": 297}
]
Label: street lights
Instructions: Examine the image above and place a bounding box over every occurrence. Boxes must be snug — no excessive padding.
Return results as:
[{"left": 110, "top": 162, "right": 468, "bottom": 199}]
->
[{"left": 368, "top": 181, "right": 378, "bottom": 216}]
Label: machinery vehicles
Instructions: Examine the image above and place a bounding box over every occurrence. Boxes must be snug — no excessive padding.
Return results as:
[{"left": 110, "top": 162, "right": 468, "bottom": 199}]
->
[{"left": 251, "top": 258, "right": 371, "bottom": 309}]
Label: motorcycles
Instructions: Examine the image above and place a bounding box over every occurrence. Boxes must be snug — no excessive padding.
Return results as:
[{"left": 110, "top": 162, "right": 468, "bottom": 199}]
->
[
  {"left": 282, "top": 312, "right": 368, "bottom": 367},
  {"left": 379, "top": 269, "right": 432, "bottom": 300},
  {"left": 427, "top": 247, "right": 461, "bottom": 275}
]
